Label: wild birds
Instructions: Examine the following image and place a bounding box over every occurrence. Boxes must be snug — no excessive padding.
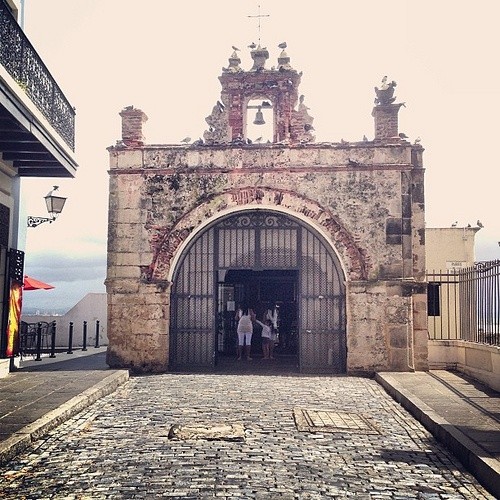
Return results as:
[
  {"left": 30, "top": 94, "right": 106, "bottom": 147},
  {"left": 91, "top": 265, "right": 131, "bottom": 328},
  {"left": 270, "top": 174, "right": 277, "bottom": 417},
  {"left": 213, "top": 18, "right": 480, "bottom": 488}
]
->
[{"left": 106, "top": 40, "right": 485, "bottom": 229}]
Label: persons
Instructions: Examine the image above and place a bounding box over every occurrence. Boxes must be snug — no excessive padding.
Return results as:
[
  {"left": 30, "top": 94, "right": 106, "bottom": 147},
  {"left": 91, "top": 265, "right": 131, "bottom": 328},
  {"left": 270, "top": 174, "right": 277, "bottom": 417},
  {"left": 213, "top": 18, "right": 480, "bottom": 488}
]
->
[
  {"left": 256, "top": 319, "right": 275, "bottom": 362},
  {"left": 263, "top": 300, "right": 280, "bottom": 360},
  {"left": 234, "top": 299, "right": 256, "bottom": 362}
]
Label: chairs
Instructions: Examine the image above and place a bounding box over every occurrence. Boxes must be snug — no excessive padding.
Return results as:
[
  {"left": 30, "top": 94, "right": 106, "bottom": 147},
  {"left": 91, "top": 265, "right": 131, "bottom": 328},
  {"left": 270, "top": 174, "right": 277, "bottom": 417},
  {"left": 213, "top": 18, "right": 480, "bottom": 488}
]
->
[
  {"left": 21, "top": 320, "right": 36, "bottom": 350},
  {"left": 41, "top": 320, "right": 55, "bottom": 349}
]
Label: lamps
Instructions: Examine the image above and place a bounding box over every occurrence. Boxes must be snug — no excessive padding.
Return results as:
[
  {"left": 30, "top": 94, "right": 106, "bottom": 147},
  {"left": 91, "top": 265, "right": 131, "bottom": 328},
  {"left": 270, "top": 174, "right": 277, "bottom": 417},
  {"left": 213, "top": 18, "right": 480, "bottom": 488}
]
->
[{"left": 27, "top": 184, "right": 68, "bottom": 228}]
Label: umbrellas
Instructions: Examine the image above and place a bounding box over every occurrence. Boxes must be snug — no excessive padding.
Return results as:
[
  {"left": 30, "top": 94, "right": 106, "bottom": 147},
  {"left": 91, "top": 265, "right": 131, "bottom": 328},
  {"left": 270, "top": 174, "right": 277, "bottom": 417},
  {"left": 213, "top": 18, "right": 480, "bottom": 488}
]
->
[{"left": 23, "top": 276, "right": 57, "bottom": 292}]
